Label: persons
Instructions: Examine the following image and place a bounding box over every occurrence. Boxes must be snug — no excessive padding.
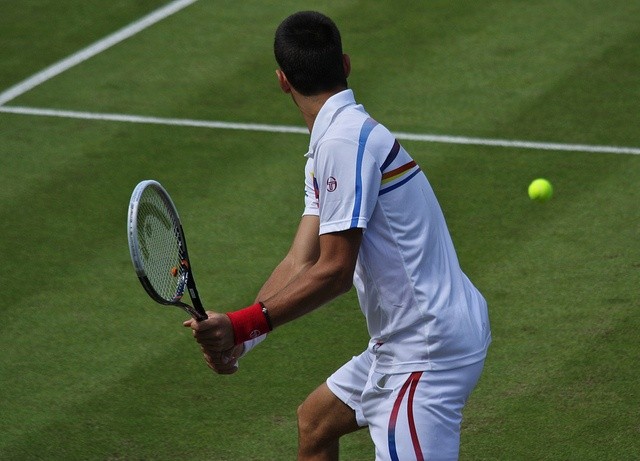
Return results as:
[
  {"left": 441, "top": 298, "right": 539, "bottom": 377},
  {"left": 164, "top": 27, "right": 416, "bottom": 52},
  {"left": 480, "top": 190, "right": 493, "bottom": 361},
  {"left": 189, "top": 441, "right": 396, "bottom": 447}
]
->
[{"left": 181, "top": 10, "right": 493, "bottom": 461}]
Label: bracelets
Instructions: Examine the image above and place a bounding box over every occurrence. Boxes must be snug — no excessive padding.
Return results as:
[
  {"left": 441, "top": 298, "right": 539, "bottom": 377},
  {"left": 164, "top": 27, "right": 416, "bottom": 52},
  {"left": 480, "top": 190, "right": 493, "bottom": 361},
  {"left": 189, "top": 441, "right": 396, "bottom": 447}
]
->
[{"left": 226, "top": 301, "right": 274, "bottom": 345}]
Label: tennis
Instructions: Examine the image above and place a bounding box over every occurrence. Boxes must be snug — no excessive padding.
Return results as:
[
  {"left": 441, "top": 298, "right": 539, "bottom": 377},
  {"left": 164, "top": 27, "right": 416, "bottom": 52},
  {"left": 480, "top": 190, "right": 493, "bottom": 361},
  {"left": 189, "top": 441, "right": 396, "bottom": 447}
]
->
[{"left": 527, "top": 177, "right": 554, "bottom": 205}]
